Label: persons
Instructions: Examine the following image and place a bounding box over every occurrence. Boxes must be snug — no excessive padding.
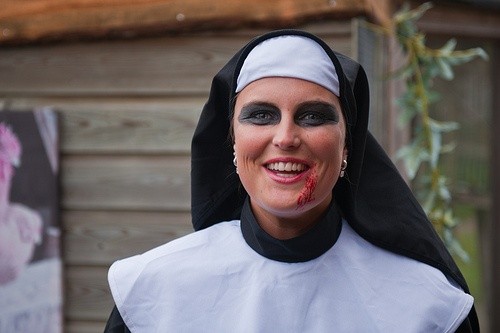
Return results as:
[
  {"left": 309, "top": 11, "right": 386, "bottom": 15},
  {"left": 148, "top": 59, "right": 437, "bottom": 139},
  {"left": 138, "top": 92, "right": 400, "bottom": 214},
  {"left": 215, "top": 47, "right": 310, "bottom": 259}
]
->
[
  {"left": 102, "top": 28, "right": 482, "bottom": 333},
  {"left": 0, "top": 120, "right": 44, "bottom": 289}
]
